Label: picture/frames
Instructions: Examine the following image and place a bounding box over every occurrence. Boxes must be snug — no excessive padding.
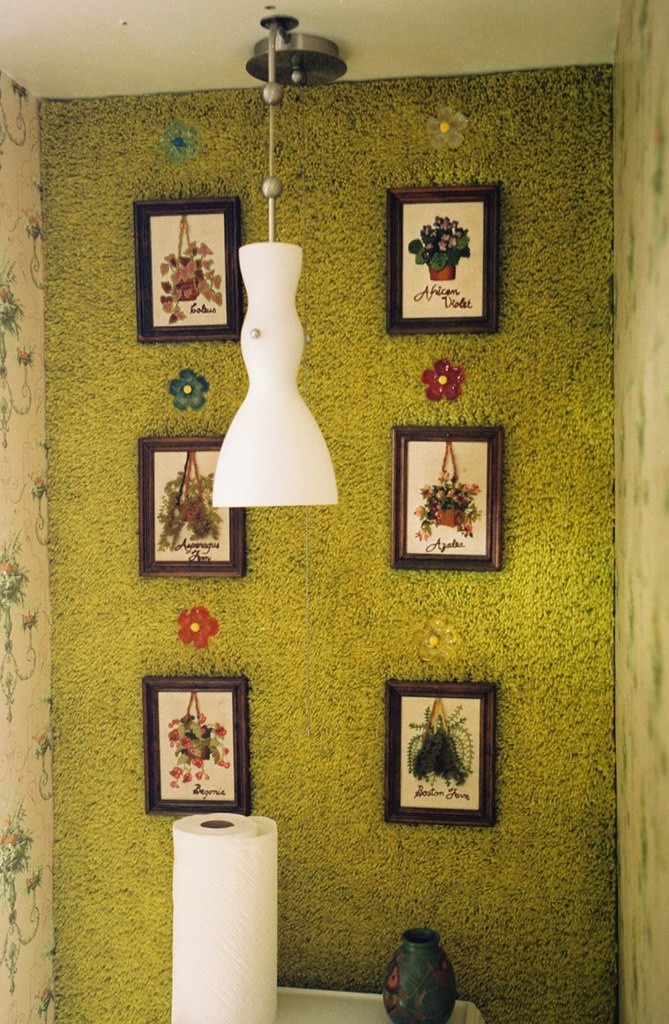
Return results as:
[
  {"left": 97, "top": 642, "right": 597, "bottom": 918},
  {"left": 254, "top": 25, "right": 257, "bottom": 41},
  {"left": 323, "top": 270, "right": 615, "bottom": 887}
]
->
[
  {"left": 132, "top": 195, "right": 244, "bottom": 343},
  {"left": 384, "top": 679, "right": 497, "bottom": 828},
  {"left": 137, "top": 436, "right": 247, "bottom": 577},
  {"left": 385, "top": 184, "right": 501, "bottom": 335},
  {"left": 389, "top": 424, "right": 504, "bottom": 572},
  {"left": 141, "top": 674, "right": 252, "bottom": 817}
]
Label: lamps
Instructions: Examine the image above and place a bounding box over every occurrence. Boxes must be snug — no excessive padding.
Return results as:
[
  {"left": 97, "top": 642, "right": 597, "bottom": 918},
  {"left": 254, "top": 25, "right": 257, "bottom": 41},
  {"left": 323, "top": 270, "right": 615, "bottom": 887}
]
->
[{"left": 212, "top": 13, "right": 338, "bottom": 509}]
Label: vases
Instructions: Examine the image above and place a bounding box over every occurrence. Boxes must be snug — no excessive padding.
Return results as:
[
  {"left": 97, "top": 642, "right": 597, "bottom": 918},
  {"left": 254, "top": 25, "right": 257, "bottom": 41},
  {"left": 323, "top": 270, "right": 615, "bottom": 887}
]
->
[{"left": 383, "top": 927, "right": 456, "bottom": 1024}]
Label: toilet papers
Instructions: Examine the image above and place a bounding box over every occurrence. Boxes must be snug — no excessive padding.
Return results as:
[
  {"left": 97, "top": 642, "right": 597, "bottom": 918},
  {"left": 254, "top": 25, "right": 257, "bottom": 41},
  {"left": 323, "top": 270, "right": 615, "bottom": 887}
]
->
[{"left": 171, "top": 809, "right": 280, "bottom": 1024}]
